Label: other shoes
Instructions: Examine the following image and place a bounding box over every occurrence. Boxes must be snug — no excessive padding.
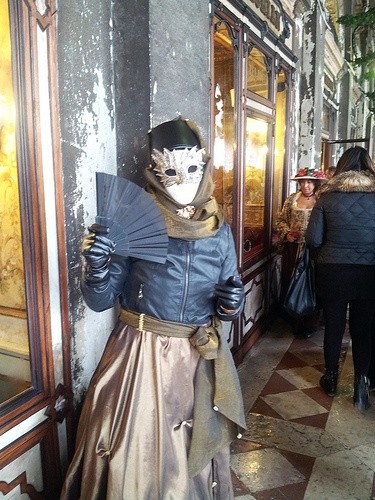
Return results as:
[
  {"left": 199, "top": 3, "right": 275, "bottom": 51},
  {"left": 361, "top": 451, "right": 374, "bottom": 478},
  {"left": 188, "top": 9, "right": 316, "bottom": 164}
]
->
[{"left": 303, "top": 326, "right": 313, "bottom": 337}]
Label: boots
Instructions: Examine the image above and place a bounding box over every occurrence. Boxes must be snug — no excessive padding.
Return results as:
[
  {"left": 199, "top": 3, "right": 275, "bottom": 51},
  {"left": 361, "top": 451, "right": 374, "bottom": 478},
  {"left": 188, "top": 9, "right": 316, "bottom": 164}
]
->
[
  {"left": 354, "top": 376, "right": 371, "bottom": 411},
  {"left": 320, "top": 369, "right": 338, "bottom": 396}
]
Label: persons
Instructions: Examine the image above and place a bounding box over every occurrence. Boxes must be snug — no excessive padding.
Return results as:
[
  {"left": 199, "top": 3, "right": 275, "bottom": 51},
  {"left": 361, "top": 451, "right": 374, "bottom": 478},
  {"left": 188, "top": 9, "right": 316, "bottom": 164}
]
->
[
  {"left": 283, "top": 146, "right": 375, "bottom": 410},
  {"left": 276, "top": 167, "right": 328, "bottom": 340},
  {"left": 59, "top": 116, "right": 248, "bottom": 500}
]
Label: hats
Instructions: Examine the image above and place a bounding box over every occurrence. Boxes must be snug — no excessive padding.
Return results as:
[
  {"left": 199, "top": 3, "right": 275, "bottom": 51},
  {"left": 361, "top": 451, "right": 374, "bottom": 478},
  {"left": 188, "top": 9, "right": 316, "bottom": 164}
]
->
[
  {"left": 149, "top": 120, "right": 201, "bottom": 166},
  {"left": 289, "top": 167, "right": 329, "bottom": 183}
]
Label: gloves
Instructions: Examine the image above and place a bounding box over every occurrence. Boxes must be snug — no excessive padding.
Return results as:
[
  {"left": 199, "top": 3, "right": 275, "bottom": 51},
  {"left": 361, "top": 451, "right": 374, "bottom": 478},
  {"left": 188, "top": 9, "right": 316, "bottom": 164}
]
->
[
  {"left": 81, "top": 224, "right": 117, "bottom": 288},
  {"left": 214, "top": 276, "right": 245, "bottom": 310}
]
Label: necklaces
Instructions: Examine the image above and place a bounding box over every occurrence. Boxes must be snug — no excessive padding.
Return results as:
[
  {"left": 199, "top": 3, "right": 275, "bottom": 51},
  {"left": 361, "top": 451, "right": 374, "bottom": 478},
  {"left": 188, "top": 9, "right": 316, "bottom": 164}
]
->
[{"left": 303, "top": 193, "right": 314, "bottom": 200}]
